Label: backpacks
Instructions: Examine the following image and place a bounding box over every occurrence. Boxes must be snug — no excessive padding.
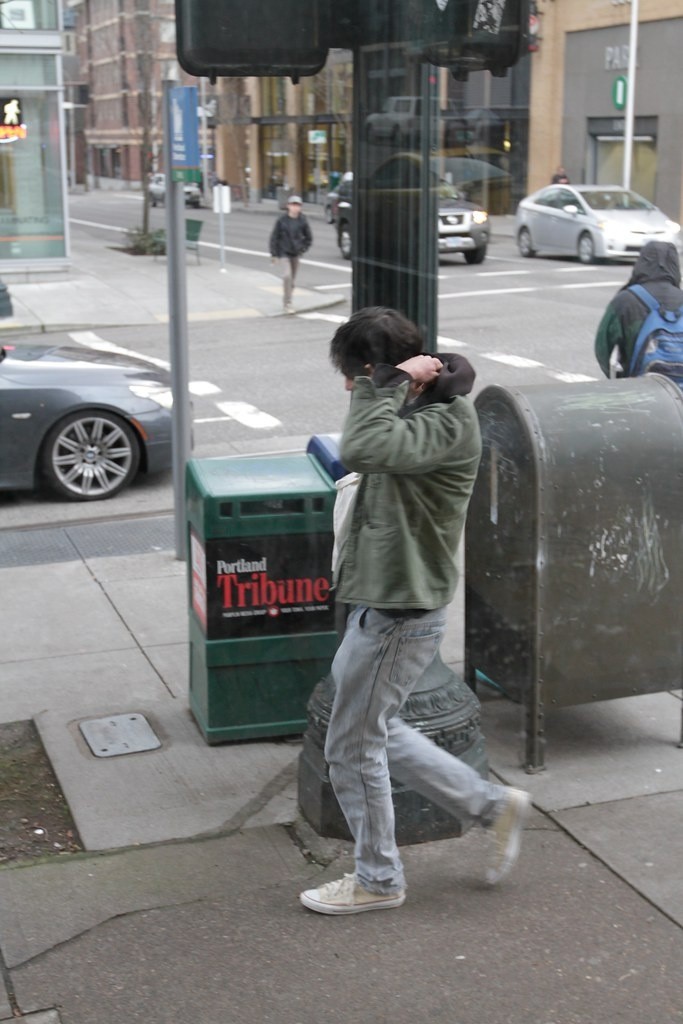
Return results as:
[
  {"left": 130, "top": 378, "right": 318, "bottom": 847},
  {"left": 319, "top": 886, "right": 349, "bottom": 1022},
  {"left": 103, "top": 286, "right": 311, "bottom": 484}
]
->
[{"left": 627, "top": 283, "right": 683, "bottom": 397}]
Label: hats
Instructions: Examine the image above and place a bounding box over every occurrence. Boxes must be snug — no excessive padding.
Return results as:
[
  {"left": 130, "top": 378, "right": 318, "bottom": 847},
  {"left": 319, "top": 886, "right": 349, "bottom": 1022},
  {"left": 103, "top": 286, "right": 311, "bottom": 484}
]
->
[{"left": 287, "top": 194, "right": 301, "bottom": 206}]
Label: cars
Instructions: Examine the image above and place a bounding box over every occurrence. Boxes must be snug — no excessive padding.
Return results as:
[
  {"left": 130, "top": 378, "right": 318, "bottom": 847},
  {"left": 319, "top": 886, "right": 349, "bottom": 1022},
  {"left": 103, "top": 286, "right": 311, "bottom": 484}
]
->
[
  {"left": 0, "top": 340, "right": 195, "bottom": 501},
  {"left": 148, "top": 173, "right": 202, "bottom": 208},
  {"left": 332, "top": 168, "right": 492, "bottom": 265},
  {"left": 515, "top": 184, "right": 683, "bottom": 265}
]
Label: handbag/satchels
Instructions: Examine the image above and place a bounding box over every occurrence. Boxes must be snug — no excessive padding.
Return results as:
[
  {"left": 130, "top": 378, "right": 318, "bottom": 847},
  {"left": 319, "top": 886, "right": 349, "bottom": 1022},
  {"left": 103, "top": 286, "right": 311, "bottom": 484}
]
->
[{"left": 329, "top": 471, "right": 364, "bottom": 591}]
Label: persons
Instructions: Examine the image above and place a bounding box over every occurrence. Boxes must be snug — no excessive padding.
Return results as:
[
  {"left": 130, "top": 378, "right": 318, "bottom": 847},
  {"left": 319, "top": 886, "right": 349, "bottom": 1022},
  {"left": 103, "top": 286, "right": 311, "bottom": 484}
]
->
[
  {"left": 299, "top": 307, "right": 532, "bottom": 917},
  {"left": 269, "top": 195, "right": 313, "bottom": 313},
  {"left": 552, "top": 166, "right": 570, "bottom": 185},
  {"left": 595, "top": 241, "right": 682, "bottom": 390}
]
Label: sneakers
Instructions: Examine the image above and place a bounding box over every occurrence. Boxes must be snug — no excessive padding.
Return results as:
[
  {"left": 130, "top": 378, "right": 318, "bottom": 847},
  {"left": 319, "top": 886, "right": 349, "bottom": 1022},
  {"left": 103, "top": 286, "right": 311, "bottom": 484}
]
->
[
  {"left": 297, "top": 872, "right": 406, "bottom": 915},
  {"left": 479, "top": 782, "right": 532, "bottom": 888},
  {"left": 285, "top": 301, "right": 296, "bottom": 314}
]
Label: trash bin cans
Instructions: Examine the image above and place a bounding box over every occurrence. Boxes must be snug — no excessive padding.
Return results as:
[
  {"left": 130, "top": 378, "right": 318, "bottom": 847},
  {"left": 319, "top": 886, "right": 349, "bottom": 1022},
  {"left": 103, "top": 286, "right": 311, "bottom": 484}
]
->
[{"left": 182, "top": 430, "right": 344, "bottom": 749}]
